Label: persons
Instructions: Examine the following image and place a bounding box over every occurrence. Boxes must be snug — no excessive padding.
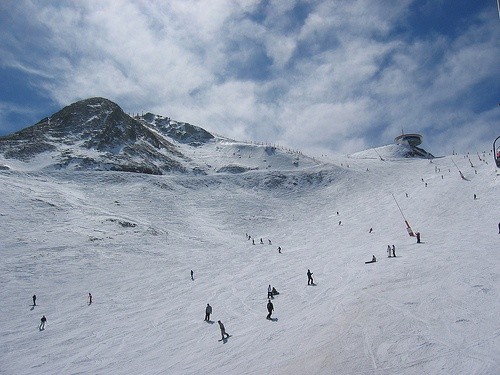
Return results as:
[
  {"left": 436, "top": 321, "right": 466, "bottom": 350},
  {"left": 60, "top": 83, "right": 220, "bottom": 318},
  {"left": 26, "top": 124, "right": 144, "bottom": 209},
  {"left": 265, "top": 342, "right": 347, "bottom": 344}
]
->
[
  {"left": 246, "top": 233, "right": 281, "bottom": 253},
  {"left": 204, "top": 303, "right": 230, "bottom": 340},
  {"left": 336, "top": 212, "right": 341, "bottom": 225},
  {"left": 365, "top": 254, "right": 376, "bottom": 264},
  {"left": 40, "top": 315, "right": 47, "bottom": 330},
  {"left": 88, "top": 293, "right": 92, "bottom": 305},
  {"left": 32, "top": 295, "right": 36, "bottom": 306},
  {"left": 300, "top": 265, "right": 318, "bottom": 287},
  {"left": 219, "top": 131, "right": 304, "bottom": 156},
  {"left": 474, "top": 194, "right": 477, "bottom": 199},
  {"left": 266, "top": 285, "right": 279, "bottom": 320},
  {"left": 387, "top": 245, "right": 396, "bottom": 257},
  {"left": 367, "top": 224, "right": 374, "bottom": 233},
  {"left": 415, "top": 232, "right": 421, "bottom": 242},
  {"left": 190, "top": 270, "right": 194, "bottom": 280}
]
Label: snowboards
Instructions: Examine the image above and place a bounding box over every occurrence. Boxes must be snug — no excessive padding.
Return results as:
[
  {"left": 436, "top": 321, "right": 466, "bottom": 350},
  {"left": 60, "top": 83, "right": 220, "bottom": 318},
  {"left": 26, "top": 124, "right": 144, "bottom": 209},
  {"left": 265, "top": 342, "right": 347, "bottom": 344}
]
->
[
  {"left": 268, "top": 318, "right": 278, "bottom": 321},
  {"left": 218, "top": 335, "right": 232, "bottom": 342}
]
